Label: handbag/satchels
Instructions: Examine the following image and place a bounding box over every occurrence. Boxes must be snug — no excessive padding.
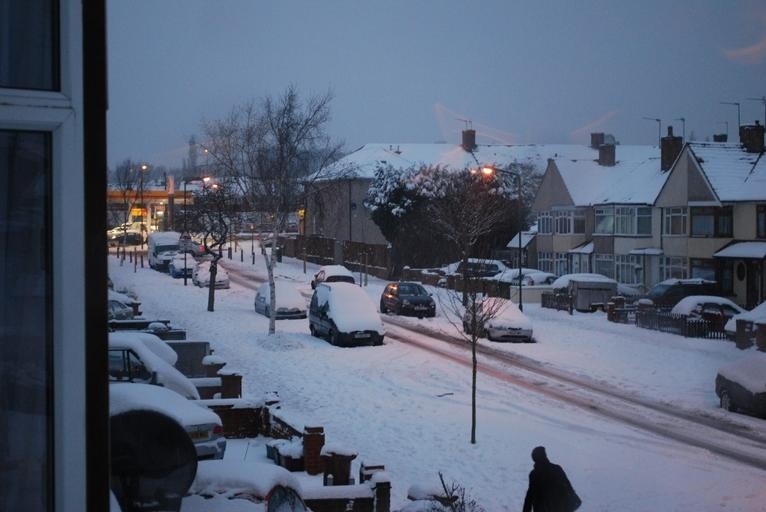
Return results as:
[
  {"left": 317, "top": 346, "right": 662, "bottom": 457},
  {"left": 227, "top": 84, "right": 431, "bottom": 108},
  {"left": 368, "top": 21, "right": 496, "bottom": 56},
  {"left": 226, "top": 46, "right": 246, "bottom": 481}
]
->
[{"left": 562, "top": 481, "right": 582, "bottom": 512}]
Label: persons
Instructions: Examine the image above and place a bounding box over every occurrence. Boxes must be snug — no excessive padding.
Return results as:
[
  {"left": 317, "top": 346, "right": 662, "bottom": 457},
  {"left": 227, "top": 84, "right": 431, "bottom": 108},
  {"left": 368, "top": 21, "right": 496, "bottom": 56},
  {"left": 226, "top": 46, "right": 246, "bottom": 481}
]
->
[{"left": 523, "top": 447, "right": 582, "bottom": 512}]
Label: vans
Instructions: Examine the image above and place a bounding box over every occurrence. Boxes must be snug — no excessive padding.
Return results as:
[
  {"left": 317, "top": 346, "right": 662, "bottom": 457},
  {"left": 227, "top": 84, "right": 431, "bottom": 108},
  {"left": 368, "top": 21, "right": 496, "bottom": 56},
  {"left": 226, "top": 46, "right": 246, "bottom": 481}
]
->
[{"left": 308, "top": 282, "right": 386, "bottom": 349}]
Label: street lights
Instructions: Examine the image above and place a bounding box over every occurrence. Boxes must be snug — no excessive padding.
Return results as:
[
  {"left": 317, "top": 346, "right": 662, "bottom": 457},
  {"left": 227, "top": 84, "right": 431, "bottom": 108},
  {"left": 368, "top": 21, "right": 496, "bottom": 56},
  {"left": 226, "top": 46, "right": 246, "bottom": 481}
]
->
[
  {"left": 251, "top": 225, "right": 254, "bottom": 252},
  {"left": 183, "top": 176, "right": 209, "bottom": 285},
  {"left": 642, "top": 117, "right": 661, "bottom": 148},
  {"left": 213, "top": 184, "right": 232, "bottom": 252},
  {"left": 720, "top": 101, "right": 740, "bottom": 134},
  {"left": 717, "top": 121, "right": 729, "bottom": 137},
  {"left": 675, "top": 117, "right": 686, "bottom": 139},
  {"left": 140, "top": 165, "right": 148, "bottom": 247},
  {"left": 748, "top": 97, "right": 765, "bottom": 131},
  {"left": 482, "top": 164, "right": 523, "bottom": 312}
]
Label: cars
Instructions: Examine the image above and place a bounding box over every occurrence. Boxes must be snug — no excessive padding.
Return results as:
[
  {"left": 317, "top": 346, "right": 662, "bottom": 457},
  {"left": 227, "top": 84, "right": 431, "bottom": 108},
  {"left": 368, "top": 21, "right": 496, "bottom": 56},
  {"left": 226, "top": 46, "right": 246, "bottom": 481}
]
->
[
  {"left": 380, "top": 282, "right": 436, "bottom": 319},
  {"left": 263, "top": 232, "right": 288, "bottom": 248},
  {"left": 108, "top": 231, "right": 230, "bottom": 466},
  {"left": 255, "top": 278, "right": 306, "bottom": 321},
  {"left": 311, "top": 265, "right": 355, "bottom": 290}
]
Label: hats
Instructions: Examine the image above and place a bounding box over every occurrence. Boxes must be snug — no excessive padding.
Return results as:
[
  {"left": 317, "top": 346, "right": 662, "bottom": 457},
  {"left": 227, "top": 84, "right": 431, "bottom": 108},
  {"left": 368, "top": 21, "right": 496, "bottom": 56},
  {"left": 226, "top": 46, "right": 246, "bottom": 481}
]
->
[{"left": 532, "top": 446, "right": 546, "bottom": 461}]
